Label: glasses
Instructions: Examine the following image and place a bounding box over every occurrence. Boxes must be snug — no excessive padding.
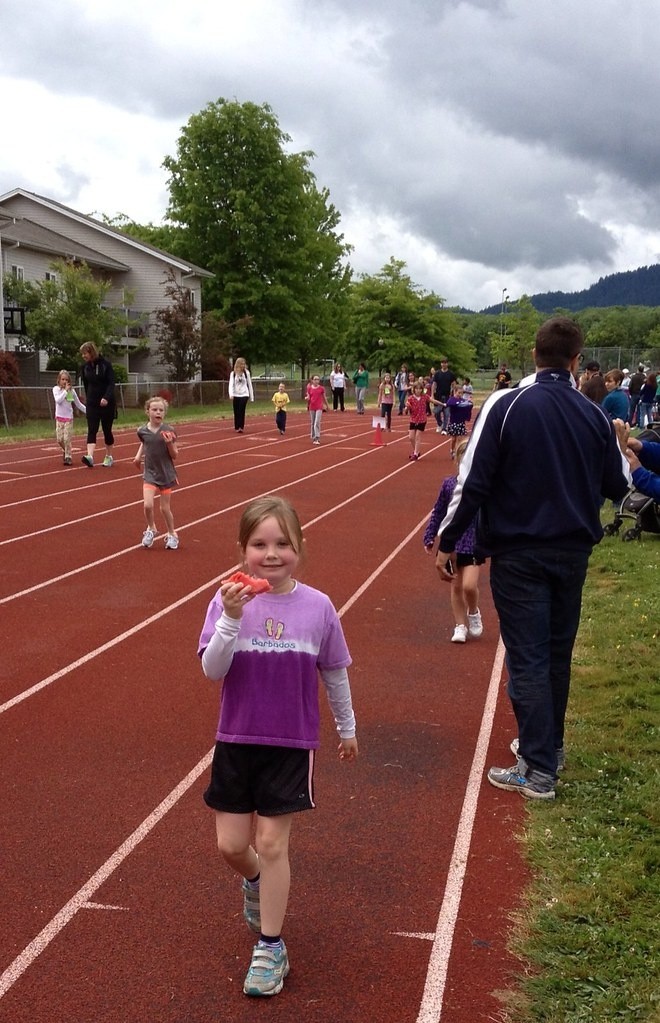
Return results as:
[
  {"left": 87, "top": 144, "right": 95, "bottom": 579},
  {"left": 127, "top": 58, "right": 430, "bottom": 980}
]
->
[{"left": 572, "top": 352, "right": 584, "bottom": 364}]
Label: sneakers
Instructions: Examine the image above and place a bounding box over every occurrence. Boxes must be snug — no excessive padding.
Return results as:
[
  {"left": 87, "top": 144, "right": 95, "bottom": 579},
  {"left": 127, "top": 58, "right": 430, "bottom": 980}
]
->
[
  {"left": 451, "top": 624, "right": 469, "bottom": 642},
  {"left": 102, "top": 455, "right": 113, "bottom": 467},
  {"left": 510, "top": 739, "right": 565, "bottom": 770},
  {"left": 243, "top": 939, "right": 291, "bottom": 996},
  {"left": 466, "top": 606, "right": 483, "bottom": 638},
  {"left": 487, "top": 758, "right": 555, "bottom": 799},
  {"left": 241, "top": 877, "right": 261, "bottom": 933},
  {"left": 163, "top": 533, "right": 179, "bottom": 549},
  {"left": 81, "top": 455, "right": 93, "bottom": 467},
  {"left": 141, "top": 527, "right": 159, "bottom": 547}
]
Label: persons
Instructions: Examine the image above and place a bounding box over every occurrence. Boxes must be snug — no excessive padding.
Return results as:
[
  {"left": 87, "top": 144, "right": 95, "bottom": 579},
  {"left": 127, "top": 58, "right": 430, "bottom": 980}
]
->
[
  {"left": 271, "top": 383, "right": 290, "bottom": 435},
  {"left": 228, "top": 358, "right": 255, "bottom": 433},
  {"left": 352, "top": 363, "right": 369, "bottom": 415},
  {"left": 80, "top": 341, "right": 118, "bottom": 468},
  {"left": 378, "top": 373, "right": 396, "bottom": 433},
  {"left": 437, "top": 317, "right": 635, "bottom": 800},
  {"left": 574, "top": 360, "right": 660, "bottom": 502},
  {"left": 196, "top": 494, "right": 359, "bottom": 996},
  {"left": 330, "top": 363, "right": 347, "bottom": 412},
  {"left": 304, "top": 375, "right": 330, "bottom": 445},
  {"left": 395, "top": 360, "right": 473, "bottom": 460},
  {"left": 133, "top": 396, "right": 179, "bottom": 550},
  {"left": 53, "top": 370, "right": 87, "bottom": 464},
  {"left": 423, "top": 437, "right": 486, "bottom": 644},
  {"left": 493, "top": 363, "right": 512, "bottom": 392}
]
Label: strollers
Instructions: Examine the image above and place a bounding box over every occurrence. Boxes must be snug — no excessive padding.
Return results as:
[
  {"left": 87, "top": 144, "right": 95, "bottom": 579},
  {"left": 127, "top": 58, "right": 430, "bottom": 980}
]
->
[{"left": 601, "top": 421, "right": 660, "bottom": 542}]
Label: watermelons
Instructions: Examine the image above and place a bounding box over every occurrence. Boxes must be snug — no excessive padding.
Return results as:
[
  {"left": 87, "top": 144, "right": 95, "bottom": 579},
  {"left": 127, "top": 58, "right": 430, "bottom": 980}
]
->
[
  {"left": 221, "top": 572, "right": 273, "bottom": 594},
  {"left": 160, "top": 431, "right": 176, "bottom": 438}
]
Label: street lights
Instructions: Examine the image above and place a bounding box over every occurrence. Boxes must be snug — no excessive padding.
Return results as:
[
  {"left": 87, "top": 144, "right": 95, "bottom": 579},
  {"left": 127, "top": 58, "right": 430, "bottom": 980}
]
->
[{"left": 498, "top": 288, "right": 509, "bottom": 371}]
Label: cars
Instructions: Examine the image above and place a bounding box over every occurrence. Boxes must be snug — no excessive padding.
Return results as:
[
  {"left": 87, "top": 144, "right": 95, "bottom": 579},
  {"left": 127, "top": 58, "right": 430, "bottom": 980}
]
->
[{"left": 252, "top": 371, "right": 287, "bottom": 380}]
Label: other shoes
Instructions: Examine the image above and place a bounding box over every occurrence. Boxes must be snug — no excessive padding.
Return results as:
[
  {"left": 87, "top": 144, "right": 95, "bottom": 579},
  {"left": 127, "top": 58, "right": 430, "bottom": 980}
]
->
[
  {"left": 441, "top": 430, "right": 447, "bottom": 435},
  {"left": 281, "top": 431, "right": 285, "bottom": 434},
  {"left": 315, "top": 437, "right": 321, "bottom": 444},
  {"left": 408, "top": 452, "right": 420, "bottom": 458},
  {"left": 436, "top": 424, "right": 443, "bottom": 432},
  {"left": 64, "top": 457, "right": 72, "bottom": 464},
  {"left": 313, "top": 439, "right": 316, "bottom": 444},
  {"left": 410, "top": 455, "right": 418, "bottom": 460},
  {"left": 237, "top": 428, "right": 243, "bottom": 432}
]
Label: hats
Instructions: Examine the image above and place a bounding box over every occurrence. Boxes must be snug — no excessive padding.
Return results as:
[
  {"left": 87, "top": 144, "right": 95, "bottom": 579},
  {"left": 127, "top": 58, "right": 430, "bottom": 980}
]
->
[
  {"left": 584, "top": 361, "right": 600, "bottom": 371},
  {"left": 622, "top": 368, "right": 629, "bottom": 374}
]
